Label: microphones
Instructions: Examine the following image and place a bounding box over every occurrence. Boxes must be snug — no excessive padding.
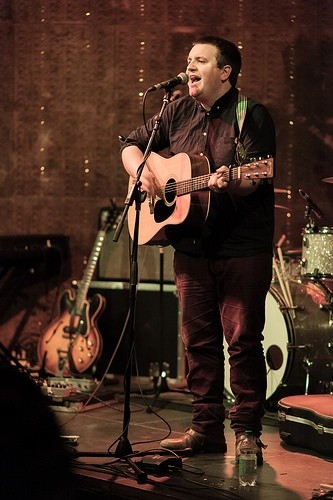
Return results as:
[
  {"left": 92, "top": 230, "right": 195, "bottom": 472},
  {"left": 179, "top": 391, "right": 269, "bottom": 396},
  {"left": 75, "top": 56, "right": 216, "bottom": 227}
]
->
[
  {"left": 299, "top": 189, "right": 325, "bottom": 221},
  {"left": 146, "top": 72, "right": 189, "bottom": 91}
]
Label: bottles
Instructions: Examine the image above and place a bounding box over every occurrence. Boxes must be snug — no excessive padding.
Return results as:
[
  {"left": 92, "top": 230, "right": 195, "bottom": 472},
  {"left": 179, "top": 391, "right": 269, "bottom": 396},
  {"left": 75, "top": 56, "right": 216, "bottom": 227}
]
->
[{"left": 239, "top": 430, "right": 257, "bottom": 487}]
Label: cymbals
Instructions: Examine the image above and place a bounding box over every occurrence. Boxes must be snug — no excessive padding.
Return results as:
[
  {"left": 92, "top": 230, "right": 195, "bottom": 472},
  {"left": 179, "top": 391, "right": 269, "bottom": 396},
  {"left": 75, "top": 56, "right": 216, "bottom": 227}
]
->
[{"left": 322, "top": 177, "right": 333, "bottom": 183}]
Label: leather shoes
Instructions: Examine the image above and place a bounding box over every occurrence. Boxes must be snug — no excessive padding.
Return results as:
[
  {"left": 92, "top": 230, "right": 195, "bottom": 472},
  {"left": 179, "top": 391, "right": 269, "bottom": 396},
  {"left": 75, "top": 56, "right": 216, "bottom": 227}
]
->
[
  {"left": 160, "top": 428, "right": 227, "bottom": 455},
  {"left": 234, "top": 432, "right": 268, "bottom": 463}
]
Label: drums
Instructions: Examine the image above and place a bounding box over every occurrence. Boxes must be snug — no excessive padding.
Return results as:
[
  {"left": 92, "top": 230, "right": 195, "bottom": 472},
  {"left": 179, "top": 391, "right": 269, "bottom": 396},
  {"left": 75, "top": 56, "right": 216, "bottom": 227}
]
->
[{"left": 224, "top": 276, "right": 333, "bottom": 405}]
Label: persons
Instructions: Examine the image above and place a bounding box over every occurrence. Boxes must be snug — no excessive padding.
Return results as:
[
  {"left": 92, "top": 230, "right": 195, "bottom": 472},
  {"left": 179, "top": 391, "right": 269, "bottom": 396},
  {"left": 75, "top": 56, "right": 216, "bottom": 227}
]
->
[{"left": 121, "top": 35, "right": 276, "bottom": 462}]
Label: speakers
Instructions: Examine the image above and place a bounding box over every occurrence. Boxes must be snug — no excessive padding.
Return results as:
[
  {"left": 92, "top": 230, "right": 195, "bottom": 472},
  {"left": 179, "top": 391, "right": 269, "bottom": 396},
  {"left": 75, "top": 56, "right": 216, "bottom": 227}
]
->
[
  {"left": 0, "top": 364, "right": 68, "bottom": 500},
  {"left": 96, "top": 206, "right": 176, "bottom": 285}
]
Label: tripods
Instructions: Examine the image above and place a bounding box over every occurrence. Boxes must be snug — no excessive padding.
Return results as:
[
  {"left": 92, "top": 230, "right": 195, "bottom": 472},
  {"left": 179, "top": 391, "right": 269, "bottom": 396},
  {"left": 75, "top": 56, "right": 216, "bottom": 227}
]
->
[{"left": 76, "top": 86, "right": 194, "bottom": 484}]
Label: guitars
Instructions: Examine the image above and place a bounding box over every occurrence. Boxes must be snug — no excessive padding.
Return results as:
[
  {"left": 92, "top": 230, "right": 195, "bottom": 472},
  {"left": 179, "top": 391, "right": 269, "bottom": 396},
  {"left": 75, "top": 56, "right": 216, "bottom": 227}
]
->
[
  {"left": 127, "top": 150, "right": 275, "bottom": 246},
  {"left": 300, "top": 225, "right": 333, "bottom": 276},
  {"left": 36, "top": 207, "right": 112, "bottom": 378}
]
